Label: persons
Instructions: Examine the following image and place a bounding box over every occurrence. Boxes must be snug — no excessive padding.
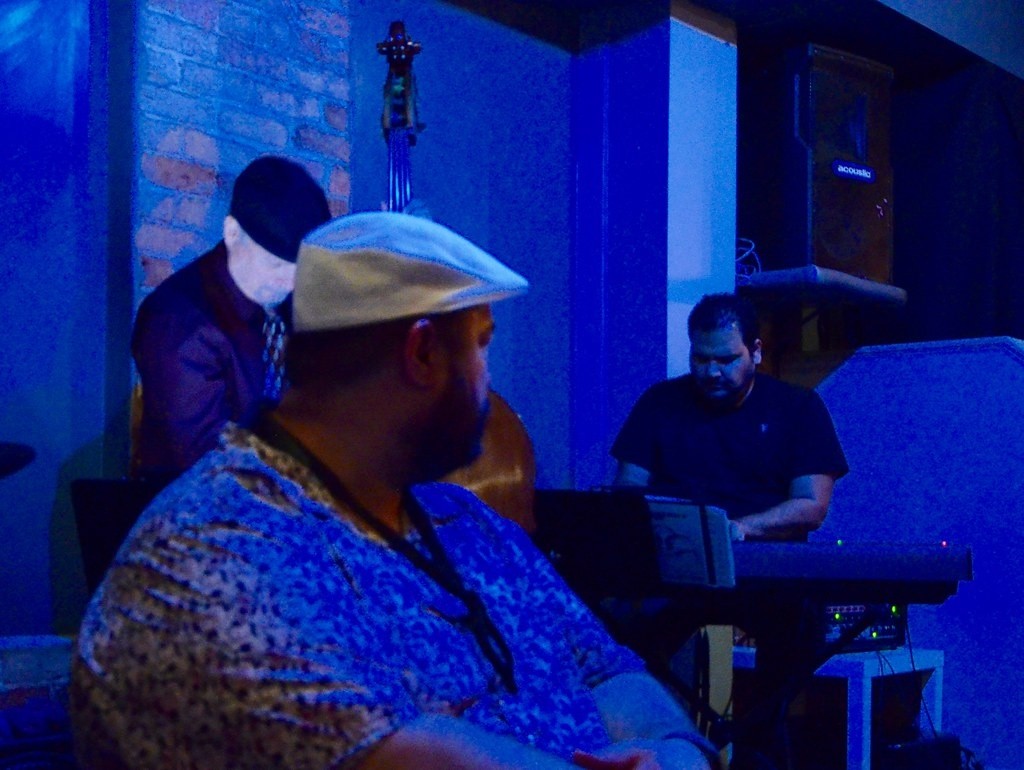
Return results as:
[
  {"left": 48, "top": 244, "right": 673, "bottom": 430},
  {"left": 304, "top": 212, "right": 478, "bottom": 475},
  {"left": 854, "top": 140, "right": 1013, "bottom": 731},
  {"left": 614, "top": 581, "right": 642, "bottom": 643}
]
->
[
  {"left": 133, "top": 158, "right": 335, "bottom": 504},
  {"left": 597, "top": 292, "right": 849, "bottom": 768},
  {"left": 68, "top": 213, "right": 715, "bottom": 768}
]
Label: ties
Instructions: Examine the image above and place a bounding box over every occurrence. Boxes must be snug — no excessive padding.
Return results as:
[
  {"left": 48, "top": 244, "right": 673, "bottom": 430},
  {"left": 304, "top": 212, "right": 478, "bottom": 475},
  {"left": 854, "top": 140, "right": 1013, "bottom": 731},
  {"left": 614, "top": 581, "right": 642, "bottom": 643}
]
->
[{"left": 263, "top": 308, "right": 291, "bottom": 399}]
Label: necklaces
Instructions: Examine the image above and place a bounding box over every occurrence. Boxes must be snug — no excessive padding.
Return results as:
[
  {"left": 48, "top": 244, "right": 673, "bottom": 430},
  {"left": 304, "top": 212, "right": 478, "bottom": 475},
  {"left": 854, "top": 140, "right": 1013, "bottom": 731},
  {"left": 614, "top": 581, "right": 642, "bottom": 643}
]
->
[{"left": 225, "top": 399, "right": 525, "bottom": 696}]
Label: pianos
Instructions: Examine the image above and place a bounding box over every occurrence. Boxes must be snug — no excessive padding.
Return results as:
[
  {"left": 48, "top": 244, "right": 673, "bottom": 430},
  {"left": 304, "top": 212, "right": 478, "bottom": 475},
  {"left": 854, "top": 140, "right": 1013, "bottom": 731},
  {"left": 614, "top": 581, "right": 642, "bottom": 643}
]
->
[{"left": 533, "top": 486, "right": 975, "bottom": 770}]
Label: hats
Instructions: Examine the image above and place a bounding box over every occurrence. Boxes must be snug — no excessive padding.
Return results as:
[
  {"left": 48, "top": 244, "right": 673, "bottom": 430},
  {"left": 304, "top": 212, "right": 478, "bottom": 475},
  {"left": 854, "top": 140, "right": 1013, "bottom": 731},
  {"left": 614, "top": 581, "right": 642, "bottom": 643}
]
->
[
  {"left": 230, "top": 156, "right": 332, "bottom": 263},
  {"left": 292, "top": 211, "right": 530, "bottom": 332}
]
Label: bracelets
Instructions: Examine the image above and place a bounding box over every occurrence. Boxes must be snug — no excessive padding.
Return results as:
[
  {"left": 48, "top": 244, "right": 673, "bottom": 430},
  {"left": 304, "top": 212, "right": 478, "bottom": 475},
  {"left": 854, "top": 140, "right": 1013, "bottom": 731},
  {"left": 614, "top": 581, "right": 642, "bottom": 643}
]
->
[{"left": 651, "top": 729, "right": 720, "bottom": 768}]
